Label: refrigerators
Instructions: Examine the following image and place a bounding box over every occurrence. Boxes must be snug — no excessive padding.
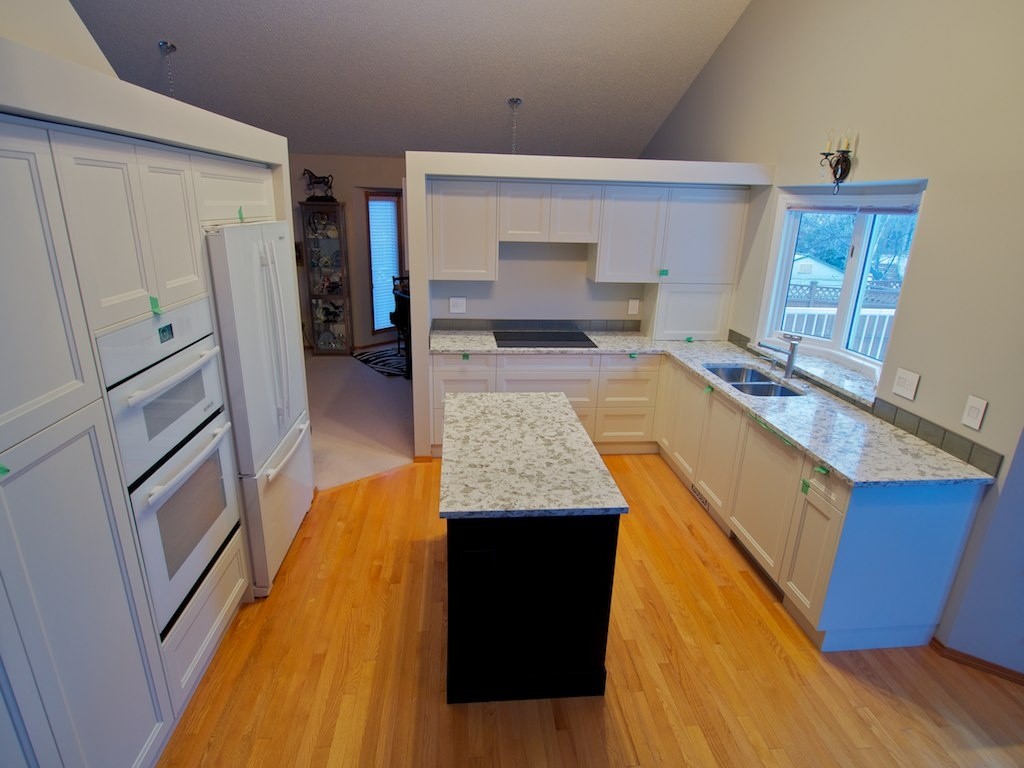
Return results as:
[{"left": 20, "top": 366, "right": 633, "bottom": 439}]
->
[{"left": 204, "top": 219, "right": 314, "bottom": 598}]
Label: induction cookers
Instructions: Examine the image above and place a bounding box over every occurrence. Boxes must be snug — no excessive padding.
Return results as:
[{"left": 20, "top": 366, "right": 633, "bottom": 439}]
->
[{"left": 492, "top": 331, "right": 598, "bottom": 348}]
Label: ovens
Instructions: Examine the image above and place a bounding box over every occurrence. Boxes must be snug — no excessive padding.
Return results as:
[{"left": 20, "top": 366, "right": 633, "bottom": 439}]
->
[{"left": 96, "top": 296, "right": 241, "bottom": 643}]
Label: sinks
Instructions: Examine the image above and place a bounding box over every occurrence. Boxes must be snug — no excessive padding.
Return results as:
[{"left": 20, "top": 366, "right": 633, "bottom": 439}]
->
[
  {"left": 705, "top": 367, "right": 776, "bottom": 383},
  {"left": 730, "top": 382, "right": 802, "bottom": 397}
]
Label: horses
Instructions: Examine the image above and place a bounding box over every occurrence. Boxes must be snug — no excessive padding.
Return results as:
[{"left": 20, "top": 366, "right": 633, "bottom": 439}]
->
[{"left": 303, "top": 168, "right": 333, "bottom": 196}]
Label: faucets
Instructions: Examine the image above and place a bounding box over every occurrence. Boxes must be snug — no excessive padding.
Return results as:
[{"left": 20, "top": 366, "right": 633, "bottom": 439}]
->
[{"left": 757, "top": 340, "right": 799, "bottom": 380}]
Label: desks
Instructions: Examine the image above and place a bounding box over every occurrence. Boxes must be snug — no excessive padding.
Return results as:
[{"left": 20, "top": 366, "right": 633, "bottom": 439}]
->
[{"left": 440, "top": 391, "right": 630, "bottom": 705}]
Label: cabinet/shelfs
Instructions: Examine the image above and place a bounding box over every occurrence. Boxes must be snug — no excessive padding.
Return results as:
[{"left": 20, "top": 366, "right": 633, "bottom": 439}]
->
[
  {"left": 431, "top": 353, "right": 497, "bottom": 445},
  {"left": 1, "top": 399, "right": 176, "bottom": 768},
  {"left": 0, "top": 122, "right": 104, "bottom": 452},
  {"left": 595, "top": 354, "right": 662, "bottom": 443},
  {"left": 658, "top": 357, "right": 742, "bottom": 512},
  {"left": 639, "top": 283, "right": 734, "bottom": 341},
  {"left": 189, "top": 149, "right": 276, "bottom": 228},
  {"left": 778, "top": 455, "right": 852, "bottom": 630},
  {"left": 497, "top": 354, "right": 600, "bottom": 443},
  {"left": 665, "top": 184, "right": 750, "bottom": 284},
  {"left": 49, "top": 129, "right": 208, "bottom": 325},
  {"left": 299, "top": 201, "right": 356, "bottom": 356},
  {"left": 730, "top": 422, "right": 806, "bottom": 583},
  {"left": 586, "top": 185, "right": 668, "bottom": 283},
  {"left": 427, "top": 176, "right": 498, "bottom": 282},
  {"left": 498, "top": 181, "right": 602, "bottom": 243}
]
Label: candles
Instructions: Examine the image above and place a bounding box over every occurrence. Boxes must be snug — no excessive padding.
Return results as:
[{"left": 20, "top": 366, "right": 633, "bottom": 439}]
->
[
  {"left": 825, "top": 129, "right": 833, "bottom": 152},
  {"left": 844, "top": 134, "right": 849, "bottom": 150}
]
